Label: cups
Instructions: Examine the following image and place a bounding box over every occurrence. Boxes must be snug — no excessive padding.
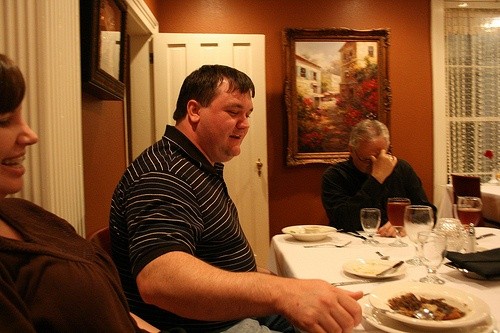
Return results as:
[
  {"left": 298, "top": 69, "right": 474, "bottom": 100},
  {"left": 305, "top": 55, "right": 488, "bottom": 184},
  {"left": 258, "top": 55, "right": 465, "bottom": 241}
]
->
[{"left": 432, "top": 217, "right": 467, "bottom": 257}]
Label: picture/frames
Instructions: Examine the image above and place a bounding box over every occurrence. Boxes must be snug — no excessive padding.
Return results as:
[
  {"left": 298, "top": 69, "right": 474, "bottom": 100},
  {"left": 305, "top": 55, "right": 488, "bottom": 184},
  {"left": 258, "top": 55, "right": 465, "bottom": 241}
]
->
[{"left": 281, "top": 25, "right": 393, "bottom": 166}]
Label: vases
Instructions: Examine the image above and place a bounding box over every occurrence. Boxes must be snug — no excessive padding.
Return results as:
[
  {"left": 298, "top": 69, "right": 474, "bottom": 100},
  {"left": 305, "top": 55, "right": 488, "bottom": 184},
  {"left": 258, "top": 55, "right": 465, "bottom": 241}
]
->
[{"left": 489, "top": 169, "right": 500, "bottom": 186}]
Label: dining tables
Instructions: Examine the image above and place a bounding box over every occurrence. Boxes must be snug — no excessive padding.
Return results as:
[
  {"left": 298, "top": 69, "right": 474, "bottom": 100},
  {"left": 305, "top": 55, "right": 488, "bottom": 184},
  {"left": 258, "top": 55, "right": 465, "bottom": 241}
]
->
[
  {"left": 270, "top": 227, "right": 500, "bottom": 333},
  {"left": 446, "top": 183, "right": 500, "bottom": 222}
]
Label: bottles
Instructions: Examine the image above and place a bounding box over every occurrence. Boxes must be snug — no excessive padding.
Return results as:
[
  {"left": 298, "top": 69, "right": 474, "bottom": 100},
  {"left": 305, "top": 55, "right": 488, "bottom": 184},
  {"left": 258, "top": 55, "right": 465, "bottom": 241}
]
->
[{"left": 467, "top": 222, "right": 476, "bottom": 254}]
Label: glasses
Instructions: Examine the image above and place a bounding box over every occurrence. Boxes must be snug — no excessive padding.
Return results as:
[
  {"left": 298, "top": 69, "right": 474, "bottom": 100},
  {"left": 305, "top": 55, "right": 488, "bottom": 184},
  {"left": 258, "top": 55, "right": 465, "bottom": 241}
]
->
[{"left": 354, "top": 149, "right": 372, "bottom": 164}]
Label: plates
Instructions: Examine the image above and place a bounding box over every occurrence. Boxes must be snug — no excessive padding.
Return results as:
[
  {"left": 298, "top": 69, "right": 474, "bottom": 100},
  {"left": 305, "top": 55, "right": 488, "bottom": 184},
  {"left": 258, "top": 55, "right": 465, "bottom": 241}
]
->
[
  {"left": 281, "top": 224, "right": 338, "bottom": 241},
  {"left": 344, "top": 255, "right": 410, "bottom": 280},
  {"left": 352, "top": 279, "right": 495, "bottom": 333}
]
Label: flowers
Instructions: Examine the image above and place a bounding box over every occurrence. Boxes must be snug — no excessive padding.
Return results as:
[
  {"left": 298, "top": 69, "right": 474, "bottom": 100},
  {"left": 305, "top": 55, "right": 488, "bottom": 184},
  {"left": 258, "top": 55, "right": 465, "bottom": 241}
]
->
[{"left": 484, "top": 150, "right": 496, "bottom": 169}]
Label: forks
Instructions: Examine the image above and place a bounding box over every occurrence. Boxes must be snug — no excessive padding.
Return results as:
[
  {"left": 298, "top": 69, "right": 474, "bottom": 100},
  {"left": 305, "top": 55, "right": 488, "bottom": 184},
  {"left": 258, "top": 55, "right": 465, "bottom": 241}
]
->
[{"left": 304, "top": 240, "right": 352, "bottom": 249}]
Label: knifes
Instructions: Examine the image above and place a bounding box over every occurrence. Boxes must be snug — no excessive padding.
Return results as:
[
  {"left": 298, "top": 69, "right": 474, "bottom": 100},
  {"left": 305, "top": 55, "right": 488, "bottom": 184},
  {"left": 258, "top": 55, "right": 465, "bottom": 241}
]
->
[{"left": 376, "top": 261, "right": 404, "bottom": 276}]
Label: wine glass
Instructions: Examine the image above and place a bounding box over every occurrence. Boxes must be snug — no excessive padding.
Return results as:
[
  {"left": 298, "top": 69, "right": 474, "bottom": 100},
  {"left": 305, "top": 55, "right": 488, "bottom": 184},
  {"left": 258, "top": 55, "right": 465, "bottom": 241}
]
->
[
  {"left": 456, "top": 195, "right": 482, "bottom": 255},
  {"left": 416, "top": 229, "right": 445, "bottom": 286},
  {"left": 360, "top": 207, "right": 381, "bottom": 245},
  {"left": 403, "top": 204, "right": 435, "bottom": 265},
  {"left": 387, "top": 197, "right": 412, "bottom": 247}
]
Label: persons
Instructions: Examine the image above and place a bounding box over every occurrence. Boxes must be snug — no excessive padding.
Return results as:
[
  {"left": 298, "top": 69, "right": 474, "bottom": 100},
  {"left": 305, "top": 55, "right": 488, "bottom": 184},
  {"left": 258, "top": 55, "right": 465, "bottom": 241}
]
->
[
  {"left": 0, "top": 54, "right": 149, "bottom": 333},
  {"left": 110, "top": 65, "right": 363, "bottom": 333},
  {"left": 321, "top": 119, "right": 438, "bottom": 237}
]
